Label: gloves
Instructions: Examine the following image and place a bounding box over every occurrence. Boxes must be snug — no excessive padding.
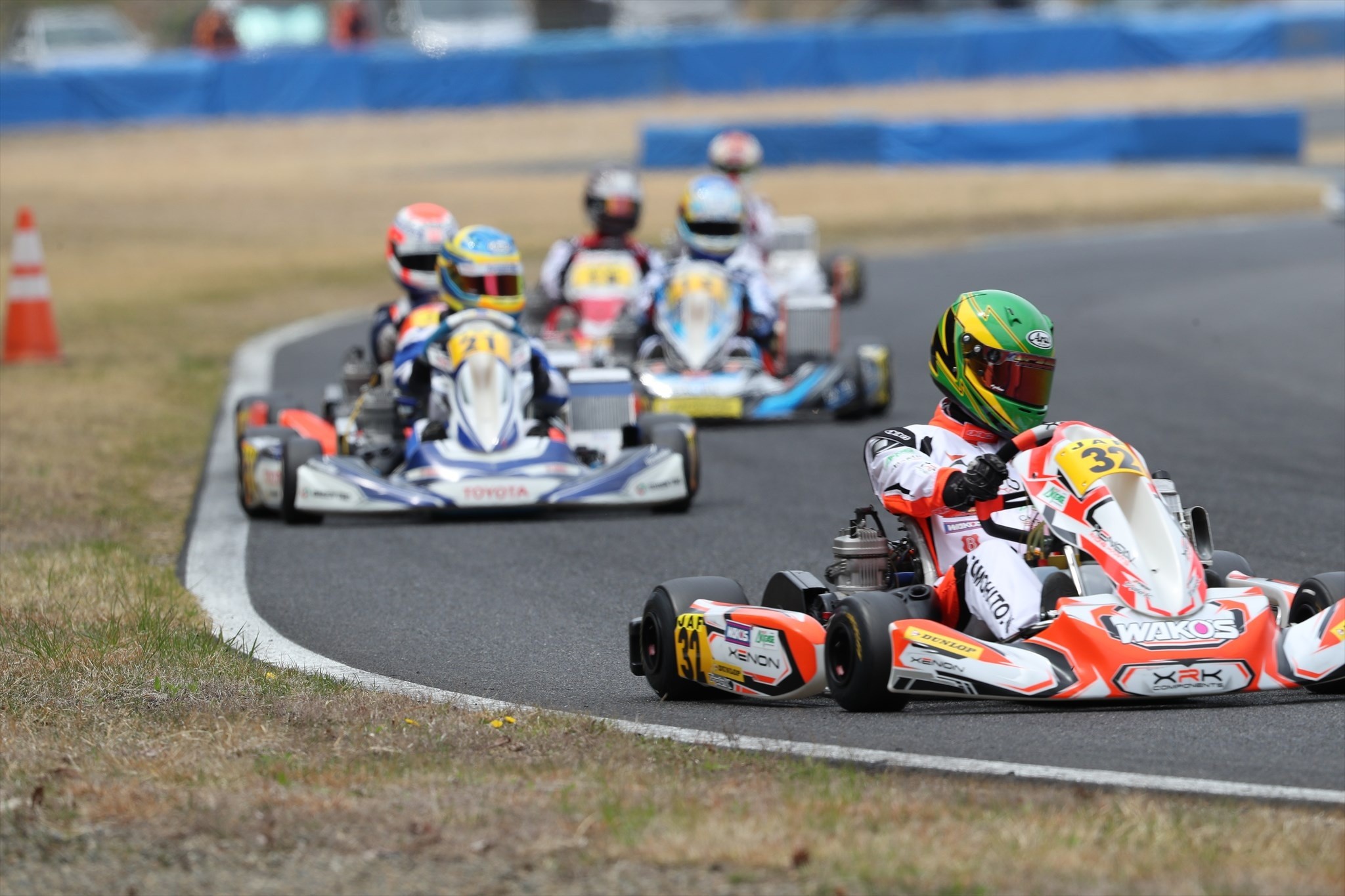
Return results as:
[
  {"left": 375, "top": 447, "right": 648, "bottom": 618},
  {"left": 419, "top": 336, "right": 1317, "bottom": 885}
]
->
[{"left": 944, "top": 453, "right": 1010, "bottom": 510}]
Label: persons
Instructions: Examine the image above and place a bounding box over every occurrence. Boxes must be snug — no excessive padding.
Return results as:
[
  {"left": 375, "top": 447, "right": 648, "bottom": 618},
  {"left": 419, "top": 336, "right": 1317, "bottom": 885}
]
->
[
  {"left": 370, "top": 201, "right": 460, "bottom": 366},
  {"left": 627, "top": 130, "right": 781, "bottom": 362},
  {"left": 865, "top": 289, "right": 1227, "bottom": 644},
  {"left": 328, "top": 0, "right": 378, "bottom": 52},
  {"left": 391, "top": 222, "right": 570, "bottom": 467},
  {"left": 518, "top": 170, "right": 672, "bottom": 364},
  {"left": 195, "top": 0, "right": 244, "bottom": 56}
]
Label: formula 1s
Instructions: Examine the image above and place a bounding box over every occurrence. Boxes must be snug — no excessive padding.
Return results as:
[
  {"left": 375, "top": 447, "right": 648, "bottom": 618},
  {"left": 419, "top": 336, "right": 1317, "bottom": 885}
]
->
[
  {"left": 527, "top": 215, "right": 897, "bottom": 421},
  {"left": 628, "top": 419, "right": 1345, "bottom": 713},
  {"left": 231, "top": 306, "right": 701, "bottom": 524}
]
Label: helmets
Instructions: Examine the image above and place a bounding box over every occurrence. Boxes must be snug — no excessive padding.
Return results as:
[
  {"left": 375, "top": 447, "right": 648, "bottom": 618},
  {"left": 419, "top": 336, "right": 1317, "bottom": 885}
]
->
[
  {"left": 929, "top": 290, "right": 1058, "bottom": 441},
  {"left": 386, "top": 204, "right": 457, "bottom": 293},
  {"left": 435, "top": 222, "right": 527, "bottom": 317},
  {"left": 708, "top": 131, "right": 766, "bottom": 168},
  {"left": 584, "top": 170, "right": 640, "bottom": 233},
  {"left": 675, "top": 171, "right": 744, "bottom": 256}
]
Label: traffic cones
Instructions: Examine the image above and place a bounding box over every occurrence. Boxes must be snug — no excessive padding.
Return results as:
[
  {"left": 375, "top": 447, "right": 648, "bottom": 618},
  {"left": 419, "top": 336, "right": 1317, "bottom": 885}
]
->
[{"left": 1, "top": 206, "right": 63, "bottom": 361}]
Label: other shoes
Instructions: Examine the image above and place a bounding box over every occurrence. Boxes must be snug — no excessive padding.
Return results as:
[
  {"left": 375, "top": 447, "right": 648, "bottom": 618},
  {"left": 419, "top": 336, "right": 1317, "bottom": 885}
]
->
[
  {"left": 1204, "top": 569, "right": 1224, "bottom": 587},
  {"left": 1040, "top": 572, "right": 1079, "bottom": 621}
]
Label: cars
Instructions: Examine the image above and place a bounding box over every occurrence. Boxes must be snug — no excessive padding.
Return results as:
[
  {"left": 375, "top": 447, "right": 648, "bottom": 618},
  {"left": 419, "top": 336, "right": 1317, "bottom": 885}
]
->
[
  {"left": 373, "top": 0, "right": 539, "bottom": 49},
  {"left": 7, "top": 3, "right": 150, "bottom": 69}
]
